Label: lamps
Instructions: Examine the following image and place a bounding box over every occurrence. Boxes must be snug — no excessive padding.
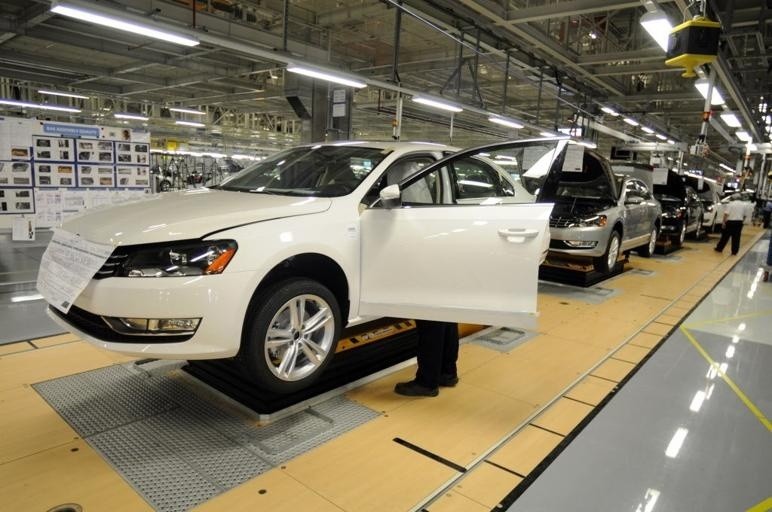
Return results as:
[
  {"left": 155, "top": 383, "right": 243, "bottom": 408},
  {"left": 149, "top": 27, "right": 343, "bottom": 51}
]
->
[
  {"left": 539, "top": 106, "right": 677, "bottom": 151},
  {"left": 0, "top": 86, "right": 206, "bottom": 128},
  {"left": 694, "top": 77, "right": 757, "bottom": 153},
  {"left": 287, "top": 62, "right": 368, "bottom": 89},
  {"left": 50, "top": 0, "right": 201, "bottom": 47},
  {"left": 638, "top": 0, "right": 674, "bottom": 53},
  {"left": 411, "top": 94, "right": 463, "bottom": 113},
  {"left": 487, "top": 116, "right": 525, "bottom": 129}
]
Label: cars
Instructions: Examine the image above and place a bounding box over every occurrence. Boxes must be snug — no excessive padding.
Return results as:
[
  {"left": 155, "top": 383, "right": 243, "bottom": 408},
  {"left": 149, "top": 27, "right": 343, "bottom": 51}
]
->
[
  {"left": 515, "top": 132, "right": 757, "bottom": 275},
  {"left": 46, "top": 136, "right": 571, "bottom": 393}
]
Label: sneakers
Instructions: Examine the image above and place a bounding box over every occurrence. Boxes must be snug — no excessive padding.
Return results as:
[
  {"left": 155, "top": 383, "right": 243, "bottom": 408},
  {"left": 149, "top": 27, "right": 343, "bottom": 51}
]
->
[{"left": 394, "top": 374, "right": 458, "bottom": 397}]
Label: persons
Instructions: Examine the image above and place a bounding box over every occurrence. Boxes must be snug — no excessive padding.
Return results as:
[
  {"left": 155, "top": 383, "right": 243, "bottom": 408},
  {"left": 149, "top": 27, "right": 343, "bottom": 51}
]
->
[
  {"left": 369, "top": 156, "right": 461, "bottom": 398},
  {"left": 712, "top": 191, "right": 746, "bottom": 256},
  {"left": 763, "top": 201, "right": 772, "bottom": 229}
]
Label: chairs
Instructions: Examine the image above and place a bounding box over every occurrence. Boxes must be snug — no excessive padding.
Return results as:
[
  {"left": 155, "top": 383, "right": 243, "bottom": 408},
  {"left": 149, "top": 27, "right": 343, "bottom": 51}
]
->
[
  {"left": 314, "top": 160, "right": 356, "bottom": 187},
  {"left": 386, "top": 162, "right": 434, "bottom": 204}
]
